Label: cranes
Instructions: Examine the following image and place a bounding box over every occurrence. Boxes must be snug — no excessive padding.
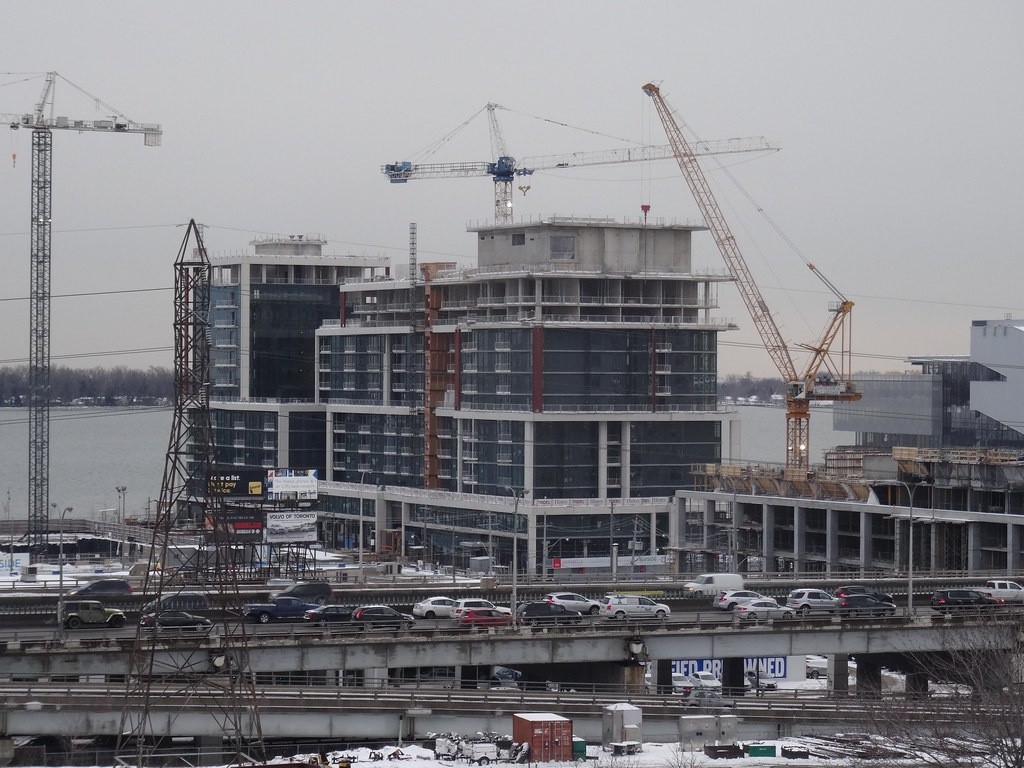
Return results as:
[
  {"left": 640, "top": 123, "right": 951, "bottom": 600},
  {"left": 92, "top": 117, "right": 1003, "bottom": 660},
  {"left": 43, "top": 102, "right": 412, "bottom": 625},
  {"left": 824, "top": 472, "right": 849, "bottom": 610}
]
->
[
  {"left": 381, "top": 100, "right": 781, "bottom": 231},
  {"left": 642, "top": 73, "right": 861, "bottom": 477},
  {"left": 4, "top": 69, "right": 162, "bottom": 565}
]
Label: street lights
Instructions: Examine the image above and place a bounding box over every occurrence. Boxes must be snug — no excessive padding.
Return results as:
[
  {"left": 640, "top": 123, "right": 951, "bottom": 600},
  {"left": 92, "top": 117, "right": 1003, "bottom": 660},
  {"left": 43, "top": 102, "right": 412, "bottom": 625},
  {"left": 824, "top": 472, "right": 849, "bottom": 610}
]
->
[
  {"left": 543, "top": 511, "right": 569, "bottom": 579},
  {"left": 49, "top": 502, "right": 74, "bottom": 633},
  {"left": 146, "top": 495, "right": 158, "bottom": 529},
  {"left": 357, "top": 467, "right": 375, "bottom": 579},
  {"left": 718, "top": 472, "right": 738, "bottom": 573},
  {"left": 504, "top": 482, "right": 532, "bottom": 630},
  {"left": 892, "top": 480, "right": 930, "bottom": 616}
]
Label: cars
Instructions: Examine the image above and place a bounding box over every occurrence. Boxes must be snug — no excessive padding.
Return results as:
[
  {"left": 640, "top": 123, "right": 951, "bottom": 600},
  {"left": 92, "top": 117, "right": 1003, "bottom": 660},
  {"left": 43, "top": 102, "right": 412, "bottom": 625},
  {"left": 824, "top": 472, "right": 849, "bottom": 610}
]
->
[
  {"left": 641, "top": 654, "right": 848, "bottom": 695},
  {"left": 59, "top": 579, "right": 1023, "bottom": 631}
]
